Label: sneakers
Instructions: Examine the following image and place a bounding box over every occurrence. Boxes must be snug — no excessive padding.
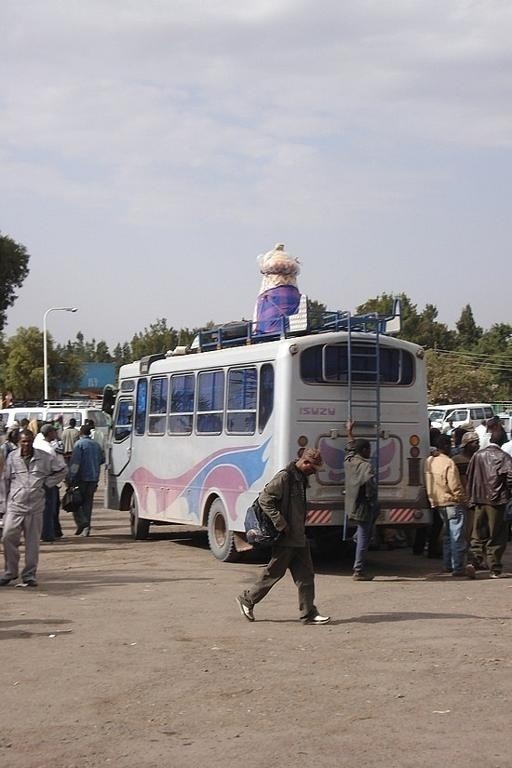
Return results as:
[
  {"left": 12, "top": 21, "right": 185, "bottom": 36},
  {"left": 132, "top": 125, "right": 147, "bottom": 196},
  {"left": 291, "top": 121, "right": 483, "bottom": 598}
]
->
[
  {"left": 352, "top": 571, "right": 375, "bottom": 581},
  {"left": 24, "top": 579, "right": 38, "bottom": 587},
  {"left": 0, "top": 573, "right": 20, "bottom": 588},
  {"left": 352, "top": 534, "right": 359, "bottom": 544},
  {"left": 443, "top": 560, "right": 511, "bottom": 579},
  {"left": 74, "top": 522, "right": 91, "bottom": 537}
]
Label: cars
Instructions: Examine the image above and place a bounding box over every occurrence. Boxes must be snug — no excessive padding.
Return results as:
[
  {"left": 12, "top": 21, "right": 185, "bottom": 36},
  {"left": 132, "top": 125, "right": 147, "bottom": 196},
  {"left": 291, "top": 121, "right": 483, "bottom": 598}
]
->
[{"left": 9, "top": 391, "right": 117, "bottom": 411}]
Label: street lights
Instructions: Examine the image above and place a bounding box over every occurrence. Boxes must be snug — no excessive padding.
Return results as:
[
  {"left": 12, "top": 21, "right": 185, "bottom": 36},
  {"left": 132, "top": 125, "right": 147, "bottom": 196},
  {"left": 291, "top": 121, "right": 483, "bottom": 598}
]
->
[{"left": 44, "top": 307, "right": 79, "bottom": 401}]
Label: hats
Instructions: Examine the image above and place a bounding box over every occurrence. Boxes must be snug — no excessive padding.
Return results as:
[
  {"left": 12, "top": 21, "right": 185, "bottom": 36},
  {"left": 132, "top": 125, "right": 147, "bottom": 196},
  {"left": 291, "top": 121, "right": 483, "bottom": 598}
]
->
[
  {"left": 457, "top": 431, "right": 480, "bottom": 449},
  {"left": 40, "top": 423, "right": 60, "bottom": 436}
]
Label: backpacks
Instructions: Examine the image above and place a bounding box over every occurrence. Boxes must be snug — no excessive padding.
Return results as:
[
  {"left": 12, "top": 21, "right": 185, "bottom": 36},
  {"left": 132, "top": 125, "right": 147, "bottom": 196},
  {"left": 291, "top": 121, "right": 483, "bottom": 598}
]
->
[{"left": 243, "top": 468, "right": 294, "bottom": 551}]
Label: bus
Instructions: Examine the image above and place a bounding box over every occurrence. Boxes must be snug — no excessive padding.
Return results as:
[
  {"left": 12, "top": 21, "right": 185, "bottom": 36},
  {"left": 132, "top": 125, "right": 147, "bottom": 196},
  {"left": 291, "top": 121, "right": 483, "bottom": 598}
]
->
[{"left": 99, "top": 305, "right": 449, "bottom": 566}]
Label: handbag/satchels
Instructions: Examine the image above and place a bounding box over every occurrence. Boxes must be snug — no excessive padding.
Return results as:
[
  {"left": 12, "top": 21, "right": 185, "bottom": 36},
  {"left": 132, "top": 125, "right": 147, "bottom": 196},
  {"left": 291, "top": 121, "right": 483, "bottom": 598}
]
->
[{"left": 61, "top": 486, "right": 82, "bottom": 515}]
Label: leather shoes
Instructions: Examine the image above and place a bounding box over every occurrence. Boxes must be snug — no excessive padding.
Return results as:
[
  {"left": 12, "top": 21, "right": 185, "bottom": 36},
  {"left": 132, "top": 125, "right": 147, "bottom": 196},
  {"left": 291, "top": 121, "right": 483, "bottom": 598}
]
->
[
  {"left": 235, "top": 593, "right": 255, "bottom": 623},
  {"left": 305, "top": 614, "right": 332, "bottom": 625}
]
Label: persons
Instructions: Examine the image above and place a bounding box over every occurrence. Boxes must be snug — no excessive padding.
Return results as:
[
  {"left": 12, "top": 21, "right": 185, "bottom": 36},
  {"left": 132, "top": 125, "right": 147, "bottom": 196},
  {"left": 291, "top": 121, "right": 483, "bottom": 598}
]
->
[
  {"left": 413, "top": 416, "right": 512, "bottom": 578},
  {"left": 342, "top": 418, "right": 381, "bottom": 581},
  {"left": 0, "top": 413, "right": 105, "bottom": 586},
  {"left": 235, "top": 448, "right": 331, "bottom": 625}
]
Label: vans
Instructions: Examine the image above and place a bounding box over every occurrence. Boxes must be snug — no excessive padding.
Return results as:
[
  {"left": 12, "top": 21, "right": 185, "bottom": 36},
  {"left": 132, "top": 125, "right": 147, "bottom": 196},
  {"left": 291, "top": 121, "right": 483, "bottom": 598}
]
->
[
  {"left": 424, "top": 399, "right": 512, "bottom": 460},
  {"left": 0, "top": 405, "right": 109, "bottom": 464}
]
